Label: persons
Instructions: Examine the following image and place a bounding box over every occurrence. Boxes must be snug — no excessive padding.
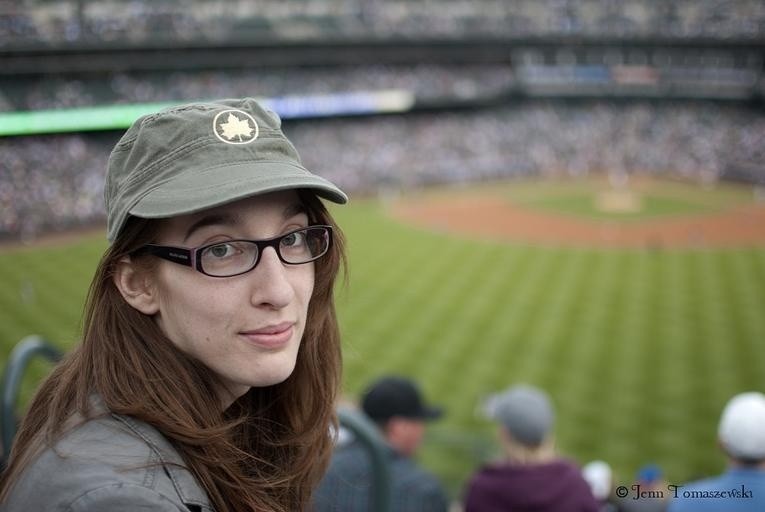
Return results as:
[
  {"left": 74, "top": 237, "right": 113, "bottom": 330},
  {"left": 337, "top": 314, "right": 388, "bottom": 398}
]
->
[
  {"left": 0, "top": 0, "right": 765, "bottom": 235},
  {"left": 580, "top": 459, "right": 621, "bottom": 510},
  {"left": 620, "top": 463, "right": 670, "bottom": 511},
  {"left": 314, "top": 374, "right": 452, "bottom": 511},
  {"left": 670, "top": 389, "right": 765, "bottom": 511},
  {"left": 1, "top": 97, "right": 352, "bottom": 510},
  {"left": 460, "top": 382, "right": 598, "bottom": 511}
]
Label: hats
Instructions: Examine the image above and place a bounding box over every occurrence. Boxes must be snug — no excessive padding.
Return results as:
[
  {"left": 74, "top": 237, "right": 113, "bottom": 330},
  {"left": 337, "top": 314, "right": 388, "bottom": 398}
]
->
[
  {"left": 719, "top": 391, "right": 765, "bottom": 461},
  {"left": 106, "top": 97, "right": 348, "bottom": 246},
  {"left": 479, "top": 381, "right": 555, "bottom": 441},
  {"left": 357, "top": 373, "right": 447, "bottom": 424}
]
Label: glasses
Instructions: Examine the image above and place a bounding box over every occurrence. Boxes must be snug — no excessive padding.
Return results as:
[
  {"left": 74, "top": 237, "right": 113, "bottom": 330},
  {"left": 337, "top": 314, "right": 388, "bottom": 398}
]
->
[{"left": 145, "top": 224, "right": 337, "bottom": 279}]
